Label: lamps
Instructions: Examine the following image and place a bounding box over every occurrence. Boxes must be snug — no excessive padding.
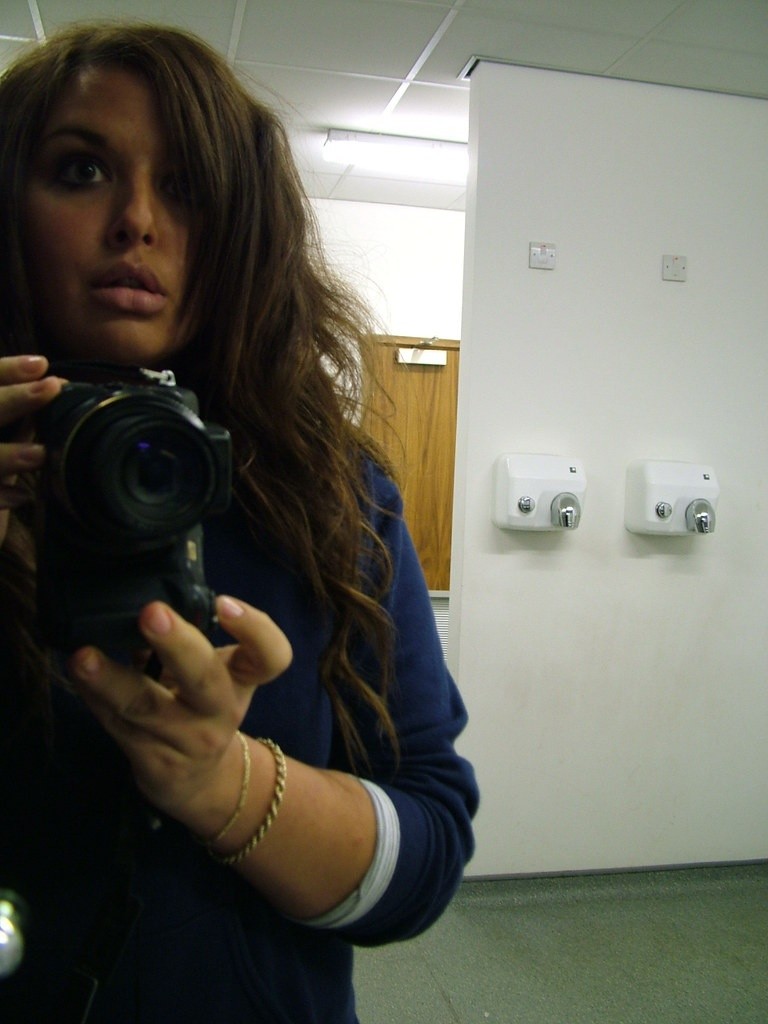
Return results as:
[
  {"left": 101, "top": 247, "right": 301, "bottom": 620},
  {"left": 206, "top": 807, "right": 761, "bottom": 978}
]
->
[{"left": 321, "top": 128, "right": 469, "bottom": 185}]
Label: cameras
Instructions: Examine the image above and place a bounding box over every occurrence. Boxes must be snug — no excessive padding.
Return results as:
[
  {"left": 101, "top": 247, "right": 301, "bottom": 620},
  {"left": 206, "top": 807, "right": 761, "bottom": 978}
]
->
[{"left": 8, "top": 381, "right": 233, "bottom": 672}]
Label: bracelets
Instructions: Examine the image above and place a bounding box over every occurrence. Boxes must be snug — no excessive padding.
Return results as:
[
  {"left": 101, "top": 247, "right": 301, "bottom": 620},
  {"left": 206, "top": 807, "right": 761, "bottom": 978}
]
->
[
  {"left": 213, "top": 730, "right": 250, "bottom": 841},
  {"left": 214, "top": 738, "right": 287, "bottom": 866}
]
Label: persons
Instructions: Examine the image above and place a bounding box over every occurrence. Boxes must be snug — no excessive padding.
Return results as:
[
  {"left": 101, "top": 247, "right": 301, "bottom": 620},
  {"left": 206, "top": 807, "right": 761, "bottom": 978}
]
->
[{"left": 2, "top": 21, "right": 478, "bottom": 1023}]
